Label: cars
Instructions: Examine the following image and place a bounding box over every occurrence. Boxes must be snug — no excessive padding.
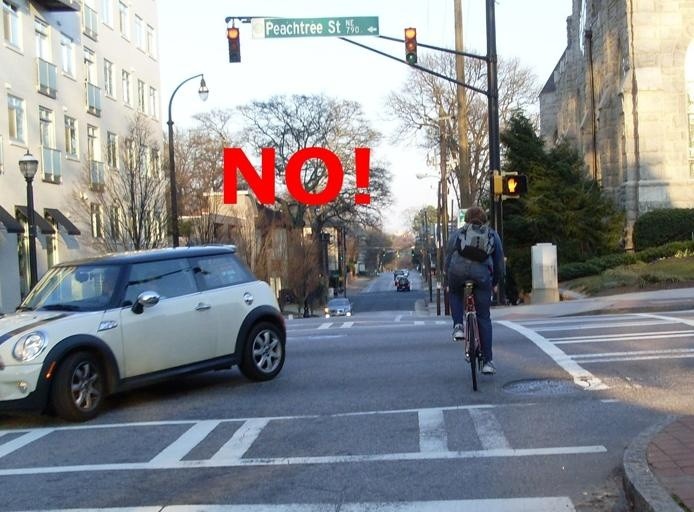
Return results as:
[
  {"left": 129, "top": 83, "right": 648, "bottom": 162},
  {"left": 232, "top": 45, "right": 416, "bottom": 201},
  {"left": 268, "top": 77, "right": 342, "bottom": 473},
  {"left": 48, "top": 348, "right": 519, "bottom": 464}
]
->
[
  {"left": 323, "top": 297, "right": 354, "bottom": 317},
  {"left": 394, "top": 267, "right": 411, "bottom": 292}
]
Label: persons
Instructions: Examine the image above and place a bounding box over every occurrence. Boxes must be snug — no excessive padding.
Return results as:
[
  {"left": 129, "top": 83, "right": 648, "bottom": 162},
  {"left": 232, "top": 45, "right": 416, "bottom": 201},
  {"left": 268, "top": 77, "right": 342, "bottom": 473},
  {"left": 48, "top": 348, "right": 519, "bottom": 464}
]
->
[
  {"left": 142, "top": 270, "right": 169, "bottom": 300},
  {"left": 441, "top": 207, "right": 504, "bottom": 376},
  {"left": 100, "top": 272, "right": 115, "bottom": 302}
]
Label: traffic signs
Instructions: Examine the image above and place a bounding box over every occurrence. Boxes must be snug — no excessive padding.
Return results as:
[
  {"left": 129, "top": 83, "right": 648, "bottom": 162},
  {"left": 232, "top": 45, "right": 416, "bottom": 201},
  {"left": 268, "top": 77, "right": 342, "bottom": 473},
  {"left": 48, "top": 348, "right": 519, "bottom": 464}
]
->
[{"left": 263, "top": 15, "right": 380, "bottom": 40}]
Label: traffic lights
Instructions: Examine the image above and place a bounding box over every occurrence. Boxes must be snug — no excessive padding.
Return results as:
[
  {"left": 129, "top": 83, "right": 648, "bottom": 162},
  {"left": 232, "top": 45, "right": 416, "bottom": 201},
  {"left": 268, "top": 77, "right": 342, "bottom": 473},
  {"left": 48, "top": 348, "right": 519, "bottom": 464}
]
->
[
  {"left": 224, "top": 26, "right": 242, "bottom": 64},
  {"left": 499, "top": 170, "right": 529, "bottom": 201},
  {"left": 402, "top": 27, "right": 419, "bottom": 65}
]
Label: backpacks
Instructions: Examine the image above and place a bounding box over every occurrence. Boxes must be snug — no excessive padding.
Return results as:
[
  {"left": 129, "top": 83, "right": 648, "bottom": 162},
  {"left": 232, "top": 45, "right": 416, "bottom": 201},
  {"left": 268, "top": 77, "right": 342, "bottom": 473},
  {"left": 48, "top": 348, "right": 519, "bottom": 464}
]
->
[{"left": 455, "top": 217, "right": 497, "bottom": 264}]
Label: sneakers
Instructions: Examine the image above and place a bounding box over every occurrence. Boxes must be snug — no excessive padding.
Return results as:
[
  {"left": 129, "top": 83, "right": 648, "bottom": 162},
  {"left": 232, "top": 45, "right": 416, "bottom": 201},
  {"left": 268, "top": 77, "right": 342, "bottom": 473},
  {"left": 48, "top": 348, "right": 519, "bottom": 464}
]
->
[
  {"left": 480, "top": 360, "right": 498, "bottom": 375},
  {"left": 452, "top": 323, "right": 466, "bottom": 342}
]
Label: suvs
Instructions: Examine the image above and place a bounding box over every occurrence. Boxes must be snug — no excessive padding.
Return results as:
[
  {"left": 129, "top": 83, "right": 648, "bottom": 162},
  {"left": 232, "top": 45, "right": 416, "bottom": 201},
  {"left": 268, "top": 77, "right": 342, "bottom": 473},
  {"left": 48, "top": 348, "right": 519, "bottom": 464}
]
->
[{"left": 0, "top": 239, "right": 288, "bottom": 424}]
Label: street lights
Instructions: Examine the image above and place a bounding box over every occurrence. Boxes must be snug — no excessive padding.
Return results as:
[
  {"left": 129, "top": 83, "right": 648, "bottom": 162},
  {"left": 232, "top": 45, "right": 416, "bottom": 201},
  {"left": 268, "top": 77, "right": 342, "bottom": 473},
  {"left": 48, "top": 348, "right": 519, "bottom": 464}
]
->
[
  {"left": 416, "top": 172, "right": 442, "bottom": 183},
  {"left": 167, "top": 71, "right": 209, "bottom": 248},
  {"left": 17, "top": 145, "right": 41, "bottom": 288}
]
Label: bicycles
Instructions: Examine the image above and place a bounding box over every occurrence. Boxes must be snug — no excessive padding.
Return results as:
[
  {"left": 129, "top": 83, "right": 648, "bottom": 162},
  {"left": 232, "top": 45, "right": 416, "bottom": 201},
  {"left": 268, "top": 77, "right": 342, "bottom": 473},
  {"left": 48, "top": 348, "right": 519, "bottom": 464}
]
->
[{"left": 460, "top": 277, "right": 486, "bottom": 392}]
